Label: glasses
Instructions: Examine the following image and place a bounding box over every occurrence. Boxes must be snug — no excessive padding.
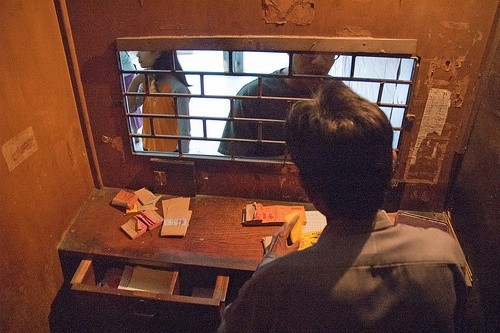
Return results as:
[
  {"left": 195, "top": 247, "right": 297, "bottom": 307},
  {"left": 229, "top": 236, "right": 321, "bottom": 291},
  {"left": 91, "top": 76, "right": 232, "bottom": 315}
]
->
[{"left": 294, "top": 49, "right": 340, "bottom": 61}]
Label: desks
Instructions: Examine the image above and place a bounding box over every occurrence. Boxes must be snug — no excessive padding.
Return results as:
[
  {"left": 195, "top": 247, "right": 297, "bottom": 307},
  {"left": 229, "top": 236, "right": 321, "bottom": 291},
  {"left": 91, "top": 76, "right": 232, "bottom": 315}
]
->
[{"left": 56, "top": 186, "right": 474, "bottom": 333}]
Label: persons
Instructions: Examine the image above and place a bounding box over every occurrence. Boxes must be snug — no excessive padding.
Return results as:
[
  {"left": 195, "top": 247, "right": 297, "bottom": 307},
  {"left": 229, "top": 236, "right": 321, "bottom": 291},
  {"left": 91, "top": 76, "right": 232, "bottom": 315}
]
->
[
  {"left": 216, "top": 52, "right": 341, "bottom": 162},
  {"left": 122, "top": 50, "right": 192, "bottom": 154},
  {"left": 118, "top": 51, "right": 145, "bottom": 144},
  {"left": 215, "top": 82, "right": 474, "bottom": 332}
]
